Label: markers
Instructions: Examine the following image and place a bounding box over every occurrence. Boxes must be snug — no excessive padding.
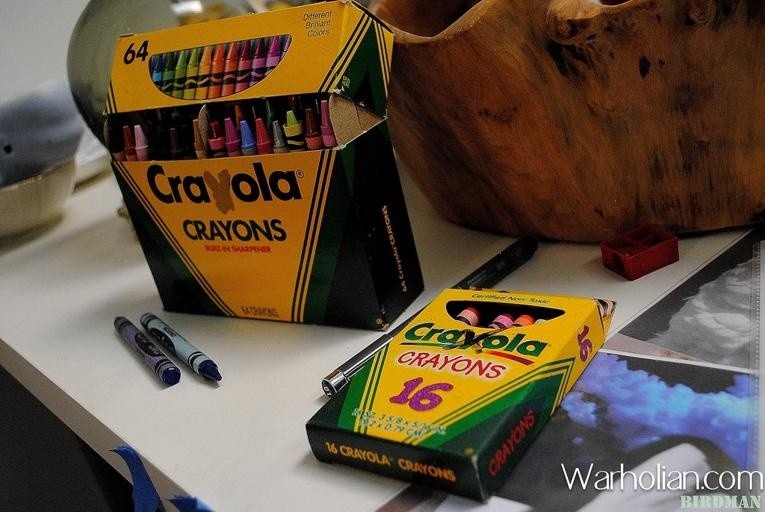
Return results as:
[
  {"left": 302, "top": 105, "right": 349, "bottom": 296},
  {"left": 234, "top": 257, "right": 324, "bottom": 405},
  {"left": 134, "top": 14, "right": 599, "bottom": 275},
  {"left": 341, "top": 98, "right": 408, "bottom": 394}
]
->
[
  {"left": 106, "top": 93, "right": 338, "bottom": 162},
  {"left": 322, "top": 234, "right": 538, "bottom": 398},
  {"left": 140, "top": 312, "right": 222, "bottom": 382},
  {"left": 113, "top": 316, "right": 180, "bottom": 386},
  {"left": 452, "top": 302, "right": 559, "bottom": 330},
  {"left": 149, "top": 34, "right": 292, "bottom": 101}
]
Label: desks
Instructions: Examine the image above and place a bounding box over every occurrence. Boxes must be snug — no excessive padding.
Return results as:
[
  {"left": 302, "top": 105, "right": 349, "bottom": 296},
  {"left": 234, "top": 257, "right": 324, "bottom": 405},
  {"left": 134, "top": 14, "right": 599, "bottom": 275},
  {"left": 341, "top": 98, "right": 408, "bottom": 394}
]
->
[{"left": 1, "top": 150, "right": 746, "bottom": 512}]
